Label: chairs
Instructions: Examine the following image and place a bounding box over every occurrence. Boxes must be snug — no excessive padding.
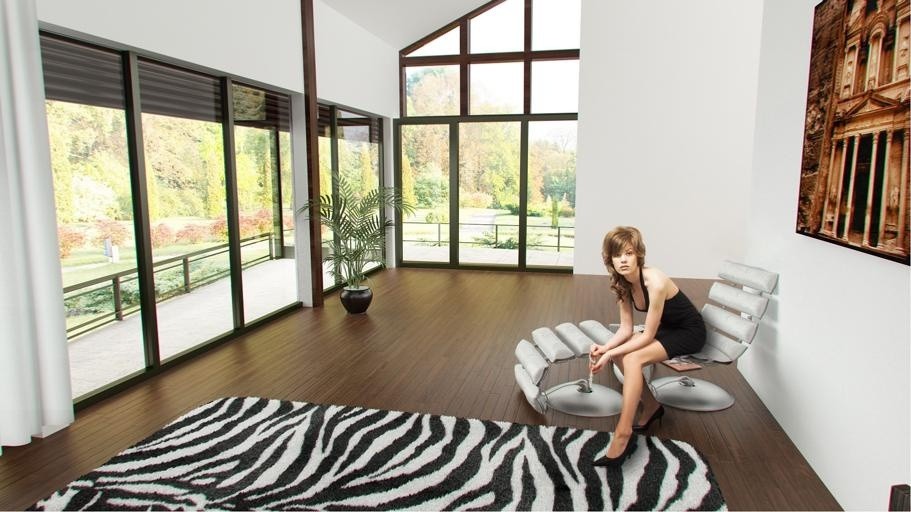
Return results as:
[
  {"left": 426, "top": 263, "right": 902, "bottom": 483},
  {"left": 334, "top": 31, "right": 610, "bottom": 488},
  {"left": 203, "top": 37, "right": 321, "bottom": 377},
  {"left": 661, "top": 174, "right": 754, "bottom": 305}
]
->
[{"left": 512, "top": 257, "right": 784, "bottom": 419}]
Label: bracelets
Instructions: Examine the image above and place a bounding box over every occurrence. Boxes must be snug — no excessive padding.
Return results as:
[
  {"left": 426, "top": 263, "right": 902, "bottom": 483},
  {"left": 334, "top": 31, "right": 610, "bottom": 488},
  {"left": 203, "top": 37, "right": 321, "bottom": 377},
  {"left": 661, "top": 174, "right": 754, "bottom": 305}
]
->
[{"left": 607, "top": 351, "right": 615, "bottom": 363}]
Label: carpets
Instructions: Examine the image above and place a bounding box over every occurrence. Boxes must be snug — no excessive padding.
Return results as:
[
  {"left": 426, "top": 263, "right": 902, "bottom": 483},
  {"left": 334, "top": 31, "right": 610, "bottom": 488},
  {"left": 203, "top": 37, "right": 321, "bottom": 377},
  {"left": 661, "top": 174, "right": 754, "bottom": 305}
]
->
[{"left": 17, "top": 393, "right": 726, "bottom": 509}]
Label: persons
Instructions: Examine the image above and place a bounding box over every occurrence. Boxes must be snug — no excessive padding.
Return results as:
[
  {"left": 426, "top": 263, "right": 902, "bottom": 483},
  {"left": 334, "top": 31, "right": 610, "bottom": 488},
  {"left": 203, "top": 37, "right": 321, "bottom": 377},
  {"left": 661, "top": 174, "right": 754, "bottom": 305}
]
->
[{"left": 586, "top": 227, "right": 706, "bottom": 465}]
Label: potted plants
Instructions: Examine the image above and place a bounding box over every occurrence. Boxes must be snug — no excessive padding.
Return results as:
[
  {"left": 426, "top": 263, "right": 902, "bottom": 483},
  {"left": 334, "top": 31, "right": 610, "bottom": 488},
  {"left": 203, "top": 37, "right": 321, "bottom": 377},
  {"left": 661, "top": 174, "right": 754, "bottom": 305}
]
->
[{"left": 295, "top": 167, "right": 415, "bottom": 312}]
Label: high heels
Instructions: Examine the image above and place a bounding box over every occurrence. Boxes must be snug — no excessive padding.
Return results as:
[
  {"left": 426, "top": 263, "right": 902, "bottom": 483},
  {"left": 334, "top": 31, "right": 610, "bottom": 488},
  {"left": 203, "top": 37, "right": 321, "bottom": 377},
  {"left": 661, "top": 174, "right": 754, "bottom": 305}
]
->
[
  {"left": 632, "top": 404, "right": 664, "bottom": 432},
  {"left": 591, "top": 432, "right": 634, "bottom": 467}
]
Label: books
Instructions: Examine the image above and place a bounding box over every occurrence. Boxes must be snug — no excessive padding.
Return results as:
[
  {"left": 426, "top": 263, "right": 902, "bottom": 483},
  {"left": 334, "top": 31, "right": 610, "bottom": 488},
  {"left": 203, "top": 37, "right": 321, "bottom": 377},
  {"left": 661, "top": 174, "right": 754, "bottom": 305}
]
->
[{"left": 660, "top": 359, "right": 701, "bottom": 372}]
